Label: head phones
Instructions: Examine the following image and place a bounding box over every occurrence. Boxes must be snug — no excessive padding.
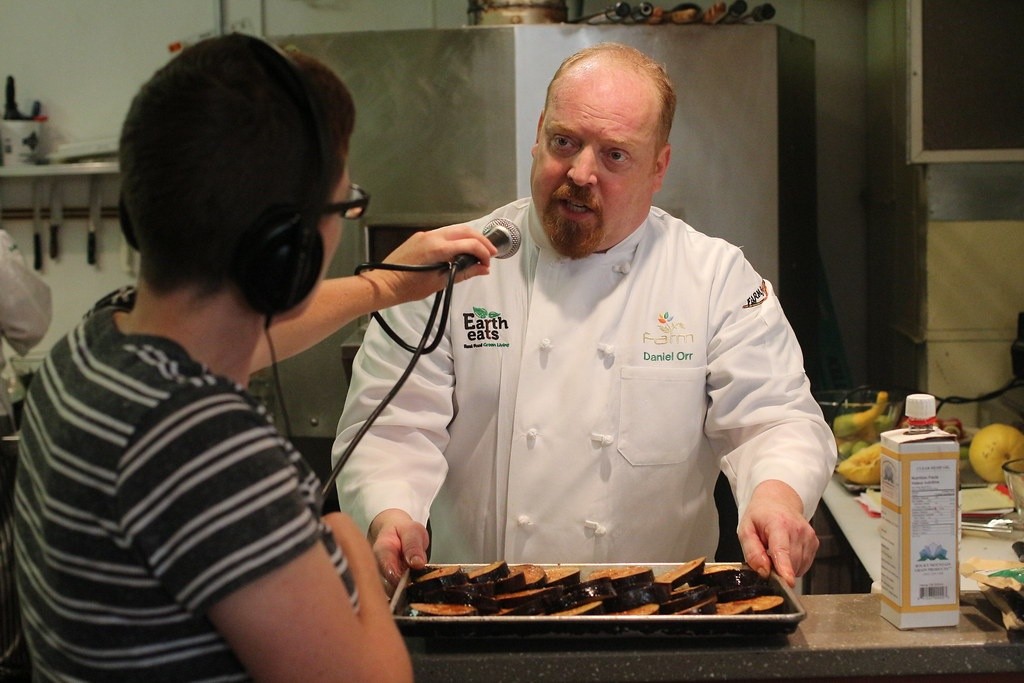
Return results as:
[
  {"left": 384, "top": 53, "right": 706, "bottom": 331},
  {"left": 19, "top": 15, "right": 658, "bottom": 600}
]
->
[{"left": 117, "top": 32, "right": 325, "bottom": 317}]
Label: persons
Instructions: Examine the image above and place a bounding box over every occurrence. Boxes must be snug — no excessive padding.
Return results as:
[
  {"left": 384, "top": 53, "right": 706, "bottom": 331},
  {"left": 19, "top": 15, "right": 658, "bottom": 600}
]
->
[
  {"left": 330, "top": 42, "right": 838, "bottom": 601},
  {"left": 0, "top": 30, "right": 497, "bottom": 683},
  {"left": 0, "top": 228, "right": 53, "bottom": 441}
]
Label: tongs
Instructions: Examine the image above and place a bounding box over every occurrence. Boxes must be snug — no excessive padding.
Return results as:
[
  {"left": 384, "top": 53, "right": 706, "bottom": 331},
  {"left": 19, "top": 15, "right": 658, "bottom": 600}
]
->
[{"left": 959, "top": 515, "right": 1015, "bottom": 538}]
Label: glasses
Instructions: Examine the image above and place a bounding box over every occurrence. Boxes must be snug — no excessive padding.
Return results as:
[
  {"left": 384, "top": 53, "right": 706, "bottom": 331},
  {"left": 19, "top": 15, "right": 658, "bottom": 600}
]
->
[{"left": 291, "top": 184, "right": 371, "bottom": 220}]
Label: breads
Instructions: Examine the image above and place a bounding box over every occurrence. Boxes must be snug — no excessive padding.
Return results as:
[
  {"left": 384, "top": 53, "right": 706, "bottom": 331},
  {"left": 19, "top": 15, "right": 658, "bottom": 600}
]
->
[{"left": 399, "top": 555, "right": 786, "bottom": 617}]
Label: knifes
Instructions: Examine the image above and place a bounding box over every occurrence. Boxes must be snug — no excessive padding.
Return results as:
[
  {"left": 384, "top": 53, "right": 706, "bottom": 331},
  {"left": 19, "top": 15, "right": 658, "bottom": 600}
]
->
[
  {"left": 31, "top": 180, "right": 42, "bottom": 270},
  {"left": 86, "top": 178, "right": 101, "bottom": 265},
  {"left": 49, "top": 179, "right": 63, "bottom": 257}
]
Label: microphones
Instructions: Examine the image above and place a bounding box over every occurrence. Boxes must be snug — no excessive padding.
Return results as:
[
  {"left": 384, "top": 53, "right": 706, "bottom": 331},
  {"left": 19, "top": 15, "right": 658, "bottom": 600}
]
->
[{"left": 451, "top": 219, "right": 521, "bottom": 272}]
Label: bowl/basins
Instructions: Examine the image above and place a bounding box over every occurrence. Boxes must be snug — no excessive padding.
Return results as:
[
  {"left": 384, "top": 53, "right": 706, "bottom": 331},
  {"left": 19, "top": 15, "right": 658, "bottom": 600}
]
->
[{"left": 812, "top": 389, "right": 890, "bottom": 427}]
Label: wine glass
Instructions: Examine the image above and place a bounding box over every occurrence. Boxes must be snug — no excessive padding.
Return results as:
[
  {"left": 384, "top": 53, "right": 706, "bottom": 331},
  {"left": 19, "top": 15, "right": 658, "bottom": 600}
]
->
[{"left": 1001, "top": 457, "right": 1024, "bottom": 530}]
